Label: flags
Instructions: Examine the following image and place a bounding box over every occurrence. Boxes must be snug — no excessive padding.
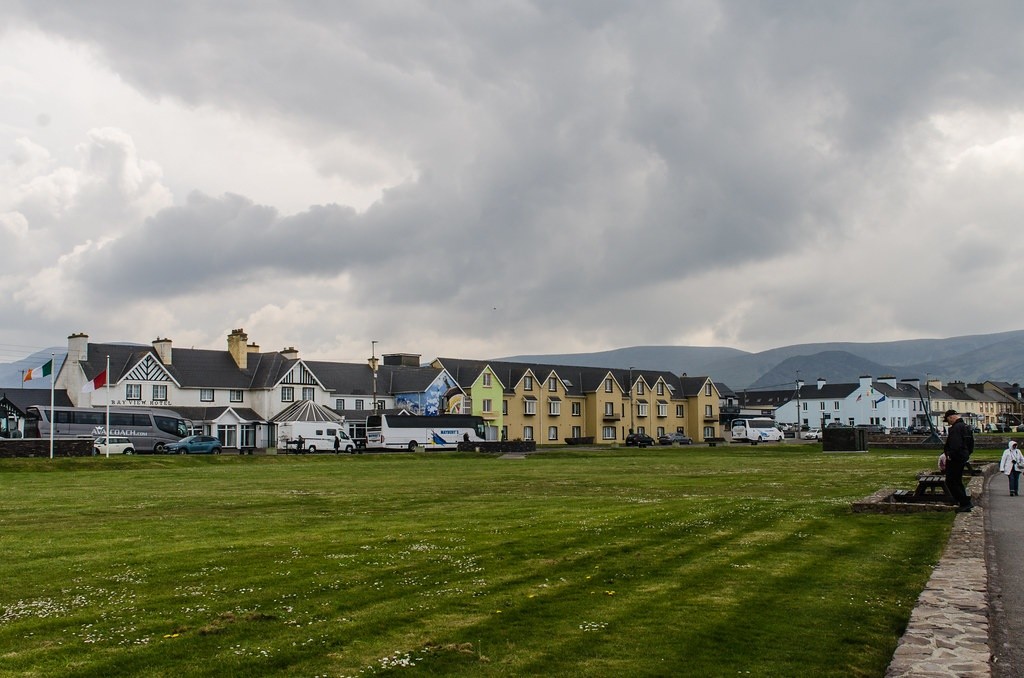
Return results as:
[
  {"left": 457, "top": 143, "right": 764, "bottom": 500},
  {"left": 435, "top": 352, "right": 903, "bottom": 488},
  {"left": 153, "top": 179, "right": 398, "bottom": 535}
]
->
[
  {"left": 876, "top": 395, "right": 885, "bottom": 403},
  {"left": 856, "top": 394, "right": 861, "bottom": 402},
  {"left": 24, "top": 359, "right": 51, "bottom": 380},
  {"left": 866, "top": 388, "right": 873, "bottom": 396},
  {"left": 82, "top": 369, "right": 106, "bottom": 394}
]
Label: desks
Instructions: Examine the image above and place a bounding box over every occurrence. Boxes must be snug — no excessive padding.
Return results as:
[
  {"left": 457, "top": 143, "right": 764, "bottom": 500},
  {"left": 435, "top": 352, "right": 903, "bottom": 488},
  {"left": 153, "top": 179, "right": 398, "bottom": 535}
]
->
[{"left": 911, "top": 476, "right": 951, "bottom": 503}]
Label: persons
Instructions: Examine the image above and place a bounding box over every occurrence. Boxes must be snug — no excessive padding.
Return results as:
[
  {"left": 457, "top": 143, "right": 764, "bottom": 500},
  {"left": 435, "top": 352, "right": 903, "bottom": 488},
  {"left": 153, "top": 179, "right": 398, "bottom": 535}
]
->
[
  {"left": 334, "top": 436, "right": 340, "bottom": 454},
  {"left": 501, "top": 430, "right": 505, "bottom": 442},
  {"left": 999, "top": 441, "right": 1024, "bottom": 496},
  {"left": 464, "top": 432, "right": 469, "bottom": 441},
  {"left": 298, "top": 434, "right": 303, "bottom": 455},
  {"left": 943, "top": 410, "right": 975, "bottom": 513}
]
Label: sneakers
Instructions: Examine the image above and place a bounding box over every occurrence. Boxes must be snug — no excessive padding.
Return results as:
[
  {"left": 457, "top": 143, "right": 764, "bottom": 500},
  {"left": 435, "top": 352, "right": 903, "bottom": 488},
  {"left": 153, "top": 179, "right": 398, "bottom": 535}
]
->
[
  {"left": 954, "top": 505, "right": 970, "bottom": 513},
  {"left": 967, "top": 502, "right": 974, "bottom": 509}
]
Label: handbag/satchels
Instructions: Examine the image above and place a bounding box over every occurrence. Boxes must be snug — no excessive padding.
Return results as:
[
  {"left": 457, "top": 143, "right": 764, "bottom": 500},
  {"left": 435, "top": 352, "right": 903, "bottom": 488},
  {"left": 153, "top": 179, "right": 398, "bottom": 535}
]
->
[{"left": 1017, "top": 462, "right": 1024, "bottom": 469}]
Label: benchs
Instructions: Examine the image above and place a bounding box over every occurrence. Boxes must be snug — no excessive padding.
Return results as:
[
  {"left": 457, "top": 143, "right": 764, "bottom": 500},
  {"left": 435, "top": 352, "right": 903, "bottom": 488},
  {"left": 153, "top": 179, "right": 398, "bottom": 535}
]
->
[
  {"left": 708, "top": 442, "right": 717, "bottom": 447},
  {"left": 750, "top": 442, "right": 757, "bottom": 445},
  {"left": 639, "top": 443, "right": 647, "bottom": 448},
  {"left": 893, "top": 490, "right": 910, "bottom": 502}
]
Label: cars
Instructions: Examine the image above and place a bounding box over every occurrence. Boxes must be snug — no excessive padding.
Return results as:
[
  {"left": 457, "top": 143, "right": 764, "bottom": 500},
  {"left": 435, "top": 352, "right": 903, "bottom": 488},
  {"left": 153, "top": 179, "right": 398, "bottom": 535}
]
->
[
  {"left": 659, "top": 432, "right": 694, "bottom": 446},
  {"left": 626, "top": 434, "right": 656, "bottom": 447},
  {"left": 804, "top": 429, "right": 821, "bottom": 440}
]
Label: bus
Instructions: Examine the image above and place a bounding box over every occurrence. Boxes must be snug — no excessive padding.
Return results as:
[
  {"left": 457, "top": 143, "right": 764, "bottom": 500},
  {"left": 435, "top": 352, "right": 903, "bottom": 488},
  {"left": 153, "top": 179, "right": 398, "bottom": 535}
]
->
[
  {"left": 730, "top": 417, "right": 782, "bottom": 443},
  {"left": 27, "top": 406, "right": 195, "bottom": 455},
  {"left": 364, "top": 413, "right": 491, "bottom": 452}
]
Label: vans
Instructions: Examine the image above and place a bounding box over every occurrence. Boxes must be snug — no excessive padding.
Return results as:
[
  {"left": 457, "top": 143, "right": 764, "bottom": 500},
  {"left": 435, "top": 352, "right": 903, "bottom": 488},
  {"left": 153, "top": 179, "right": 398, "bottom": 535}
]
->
[{"left": 277, "top": 421, "right": 358, "bottom": 454}]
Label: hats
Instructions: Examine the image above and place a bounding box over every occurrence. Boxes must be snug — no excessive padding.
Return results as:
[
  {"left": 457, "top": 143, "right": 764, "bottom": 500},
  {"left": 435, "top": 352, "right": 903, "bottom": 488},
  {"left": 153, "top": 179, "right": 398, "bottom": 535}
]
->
[{"left": 940, "top": 409, "right": 958, "bottom": 422}]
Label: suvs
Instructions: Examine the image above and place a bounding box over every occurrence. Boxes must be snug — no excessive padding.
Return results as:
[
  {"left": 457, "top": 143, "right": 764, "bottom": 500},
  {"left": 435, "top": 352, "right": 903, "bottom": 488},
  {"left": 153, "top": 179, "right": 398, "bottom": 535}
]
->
[
  {"left": 162, "top": 435, "right": 223, "bottom": 455},
  {"left": 92, "top": 436, "right": 136, "bottom": 456}
]
